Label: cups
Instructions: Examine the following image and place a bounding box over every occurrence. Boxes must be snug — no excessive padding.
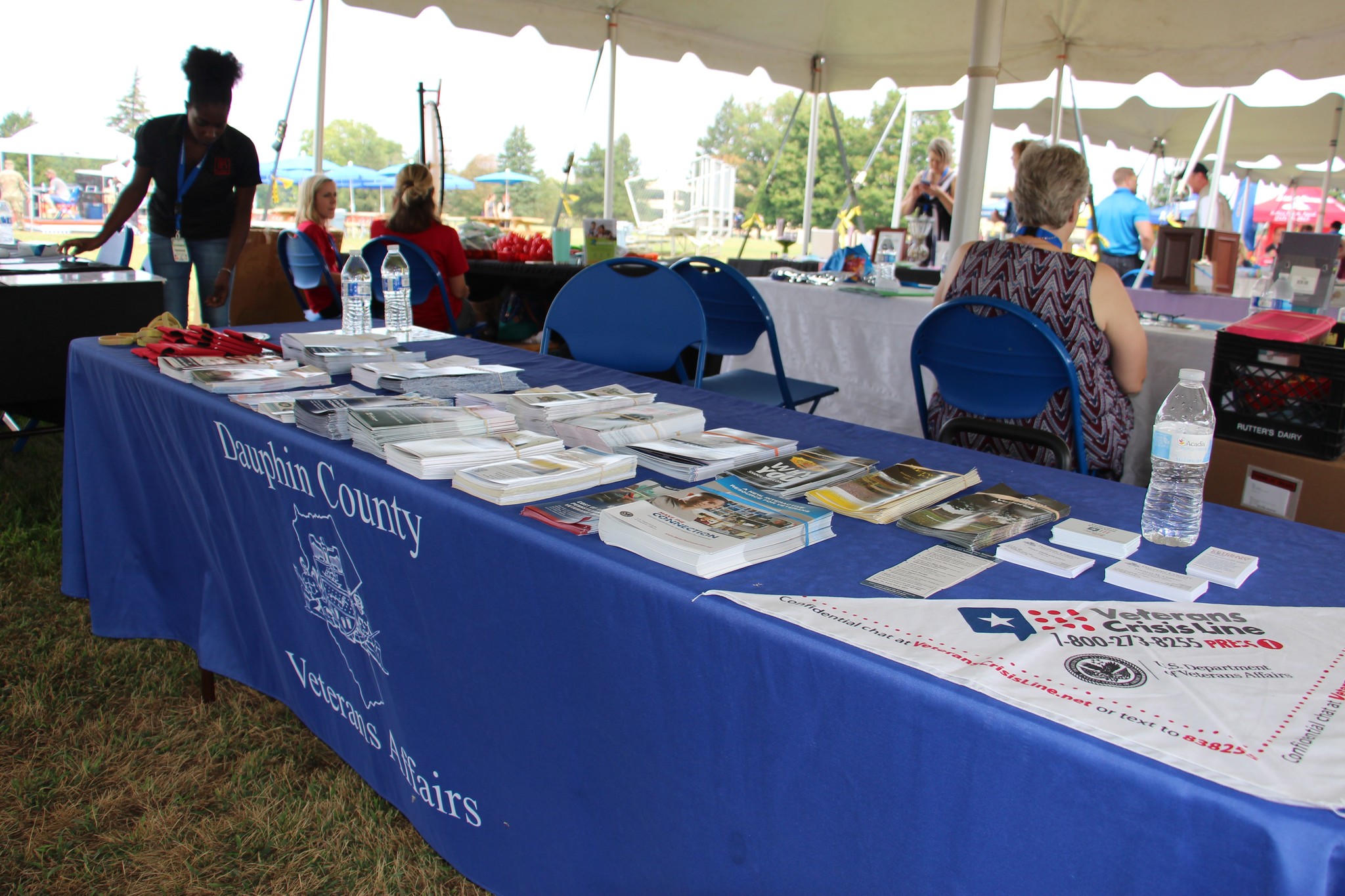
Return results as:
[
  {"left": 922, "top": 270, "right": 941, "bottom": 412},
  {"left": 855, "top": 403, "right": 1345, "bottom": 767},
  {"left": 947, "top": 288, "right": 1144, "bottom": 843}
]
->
[{"left": 551, "top": 228, "right": 571, "bottom": 266}]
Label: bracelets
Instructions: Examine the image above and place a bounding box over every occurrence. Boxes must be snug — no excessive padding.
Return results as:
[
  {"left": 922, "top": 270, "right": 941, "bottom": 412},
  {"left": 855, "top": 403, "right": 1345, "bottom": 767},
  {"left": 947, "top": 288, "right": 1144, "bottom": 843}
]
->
[
  {"left": 218, "top": 267, "right": 231, "bottom": 275},
  {"left": 103, "top": 221, "right": 124, "bottom": 233}
]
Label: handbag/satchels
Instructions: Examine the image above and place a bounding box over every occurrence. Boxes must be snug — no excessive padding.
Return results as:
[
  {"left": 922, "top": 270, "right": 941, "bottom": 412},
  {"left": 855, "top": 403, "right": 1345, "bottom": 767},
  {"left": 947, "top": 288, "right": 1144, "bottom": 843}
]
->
[{"left": 820, "top": 244, "right": 874, "bottom": 283}]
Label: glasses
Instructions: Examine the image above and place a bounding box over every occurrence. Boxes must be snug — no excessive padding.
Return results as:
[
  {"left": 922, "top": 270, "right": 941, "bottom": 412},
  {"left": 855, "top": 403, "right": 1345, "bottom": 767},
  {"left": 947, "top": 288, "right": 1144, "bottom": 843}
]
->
[
  {"left": 925, "top": 156, "right": 941, "bottom": 164},
  {"left": 1079, "top": 198, "right": 1088, "bottom": 213}
]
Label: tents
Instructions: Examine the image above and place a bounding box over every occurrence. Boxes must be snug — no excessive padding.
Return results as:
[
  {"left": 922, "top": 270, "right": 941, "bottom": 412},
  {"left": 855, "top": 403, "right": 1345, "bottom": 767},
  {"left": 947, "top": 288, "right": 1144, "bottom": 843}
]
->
[
  {"left": 0, "top": 123, "right": 135, "bottom": 234},
  {"left": 831, "top": 64, "right": 1345, "bottom": 267}
]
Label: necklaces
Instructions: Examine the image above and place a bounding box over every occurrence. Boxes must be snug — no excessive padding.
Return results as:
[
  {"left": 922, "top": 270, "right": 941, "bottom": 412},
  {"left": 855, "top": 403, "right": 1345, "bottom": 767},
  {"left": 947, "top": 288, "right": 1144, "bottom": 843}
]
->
[{"left": 292, "top": 175, "right": 384, "bottom": 319}]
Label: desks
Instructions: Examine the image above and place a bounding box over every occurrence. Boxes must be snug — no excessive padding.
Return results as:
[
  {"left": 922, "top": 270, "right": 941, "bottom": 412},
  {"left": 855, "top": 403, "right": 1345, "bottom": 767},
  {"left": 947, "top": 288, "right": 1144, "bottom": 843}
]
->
[
  {"left": 62, "top": 319, "right": 1345, "bottom": 896},
  {"left": 728, "top": 259, "right": 819, "bottom": 277},
  {"left": 1126, "top": 287, "right": 1345, "bottom": 323},
  {"left": 0, "top": 253, "right": 164, "bottom": 439},
  {"left": 338, "top": 252, "right": 723, "bottom": 386},
  {"left": 720, "top": 270, "right": 1300, "bottom": 492}
]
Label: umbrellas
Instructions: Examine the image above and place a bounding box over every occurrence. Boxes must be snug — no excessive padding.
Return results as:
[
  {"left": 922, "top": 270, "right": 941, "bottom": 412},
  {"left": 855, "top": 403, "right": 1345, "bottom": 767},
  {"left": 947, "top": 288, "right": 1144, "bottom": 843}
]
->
[
  {"left": 259, "top": 151, "right": 476, "bottom": 215},
  {"left": 474, "top": 168, "right": 540, "bottom": 219}
]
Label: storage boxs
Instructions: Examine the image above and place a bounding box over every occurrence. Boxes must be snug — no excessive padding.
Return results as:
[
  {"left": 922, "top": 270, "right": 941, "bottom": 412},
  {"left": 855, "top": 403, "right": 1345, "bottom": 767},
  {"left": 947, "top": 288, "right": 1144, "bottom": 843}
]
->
[
  {"left": 1202, "top": 309, "right": 1345, "bottom": 533},
  {"left": 1153, "top": 226, "right": 1240, "bottom": 296},
  {"left": 83, "top": 202, "right": 103, "bottom": 219}
]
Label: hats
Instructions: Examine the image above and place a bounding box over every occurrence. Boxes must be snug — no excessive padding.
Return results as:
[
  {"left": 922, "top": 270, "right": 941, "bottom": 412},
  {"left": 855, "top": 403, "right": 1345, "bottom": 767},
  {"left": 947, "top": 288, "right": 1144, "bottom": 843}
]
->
[{"left": 1174, "top": 163, "right": 1208, "bottom": 179}]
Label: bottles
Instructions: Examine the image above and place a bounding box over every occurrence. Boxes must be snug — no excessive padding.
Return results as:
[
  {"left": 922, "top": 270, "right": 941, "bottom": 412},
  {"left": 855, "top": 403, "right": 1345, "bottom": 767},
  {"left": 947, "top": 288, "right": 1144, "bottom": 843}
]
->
[
  {"left": 340, "top": 249, "right": 372, "bottom": 336},
  {"left": 874, "top": 232, "right": 896, "bottom": 291},
  {"left": 1248, "top": 268, "right": 1277, "bottom": 316},
  {"left": 1266, "top": 272, "right": 1295, "bottom": 312},
  {"left": 940, "top": 249, "right": 949, "bottom": 279},
  {"left": 0, "top": 201, "right": 14, "bottom": 245},
  {"left": 1140, "top": 368, "right": 1216, "bottom": 548},
  {"left": 380, "top": 245, "right": 413, "bottom": 332}
]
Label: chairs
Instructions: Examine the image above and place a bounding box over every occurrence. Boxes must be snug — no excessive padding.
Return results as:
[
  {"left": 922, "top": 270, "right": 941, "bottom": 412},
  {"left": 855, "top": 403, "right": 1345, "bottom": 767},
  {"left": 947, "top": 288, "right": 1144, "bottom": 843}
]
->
[
  {"left": 910, "top": 296, "right": 1098, "bottom": 476},
  {"left": 276, "top": 228, "right": 343, "bottom": 319},
  {"left": 539, "top": 256, "right": 707, "bottom": 388},
  {"left": 12, "top": 225, "right": 134, "bottom": 452},
  {"left": 668, "top": 257, "right": 839, "bottom": 415},
  {"left": 1120, "top": 268, "right": 1154, "bottom": 288},
  {"left": 55, "top": 187, "right": 79, "bottom": 220},
  {"left": 362, "top": 235, "right": 489, "bottom": 339}
]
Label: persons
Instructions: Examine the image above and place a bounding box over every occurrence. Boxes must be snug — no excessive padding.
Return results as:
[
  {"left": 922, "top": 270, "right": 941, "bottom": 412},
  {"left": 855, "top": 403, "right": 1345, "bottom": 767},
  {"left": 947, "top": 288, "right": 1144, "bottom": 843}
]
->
[
  {"left": 1264, "top": 226, "right": 1286, "bottom": 258},
  {"left": 56, "top": 45, "right": 262, "bottom": 329},
  {"left": 370, "top": 163, "right": 476, "bottom": 338},
  {"left": 1327, "top": 221, "right": 1343, "bottom": 234},
  {"left": 1175, "top": 162, "right": 1233, "bottom": 234},
  {"left": 484, "top": 192, "right": 513, "bottom": 234},
  {"left": 1300, "top": 225, "right": 1314, "bottom": 232},
  {"left": 927, "top": 139, "right": 1149, "bottom": 481},
  {"left": 585, "top": 221, "right": 612, "bottom": 239},
  {"left": 991, "top": 139, "right": 1034, "bottom": 234},
  {"left": 43, "top": 169, "right": 70, "bottom": 220},
  {"left": 901, "top": 137, "right": 958, "bottom": 268},
  {"left": 1087, "top": 167, "right": 1154, "bottom": 278},
  {"left": 0, "top": 159, "right": 27, "bottom": 230}
]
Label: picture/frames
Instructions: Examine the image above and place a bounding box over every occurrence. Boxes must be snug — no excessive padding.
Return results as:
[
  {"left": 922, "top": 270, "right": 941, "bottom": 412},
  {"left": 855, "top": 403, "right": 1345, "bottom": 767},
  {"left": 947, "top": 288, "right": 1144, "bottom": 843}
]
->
[{"left": 870, "top": 228, "right": 907, "bottom": 262}]
